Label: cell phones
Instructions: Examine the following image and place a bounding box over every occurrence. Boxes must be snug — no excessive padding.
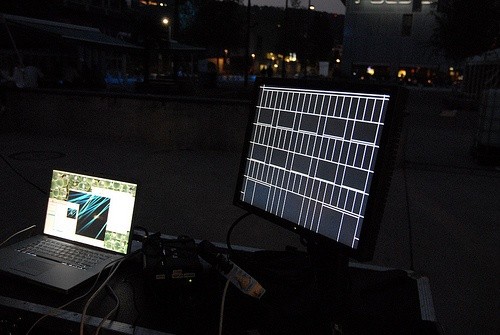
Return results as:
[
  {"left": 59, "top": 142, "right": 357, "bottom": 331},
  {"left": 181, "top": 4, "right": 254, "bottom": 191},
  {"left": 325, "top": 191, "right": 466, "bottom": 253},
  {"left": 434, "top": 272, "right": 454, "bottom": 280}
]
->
[{"left": 112, "top": 263, "right": 139, "bottom": 285}]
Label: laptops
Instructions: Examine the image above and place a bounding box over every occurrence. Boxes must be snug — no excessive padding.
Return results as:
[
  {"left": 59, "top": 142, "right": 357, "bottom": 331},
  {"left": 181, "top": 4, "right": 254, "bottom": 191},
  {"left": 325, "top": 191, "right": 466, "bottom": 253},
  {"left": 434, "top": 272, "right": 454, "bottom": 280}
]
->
[{"left": 0, "top": 167, "right": 141, "bottom": 294}]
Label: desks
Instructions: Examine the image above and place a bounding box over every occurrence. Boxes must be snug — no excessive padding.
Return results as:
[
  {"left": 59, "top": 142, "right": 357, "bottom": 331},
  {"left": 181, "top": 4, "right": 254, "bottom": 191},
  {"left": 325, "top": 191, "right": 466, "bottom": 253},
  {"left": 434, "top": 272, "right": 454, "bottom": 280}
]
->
[{"left": 0, "top": 231, "right": 449, "bottom": 335}]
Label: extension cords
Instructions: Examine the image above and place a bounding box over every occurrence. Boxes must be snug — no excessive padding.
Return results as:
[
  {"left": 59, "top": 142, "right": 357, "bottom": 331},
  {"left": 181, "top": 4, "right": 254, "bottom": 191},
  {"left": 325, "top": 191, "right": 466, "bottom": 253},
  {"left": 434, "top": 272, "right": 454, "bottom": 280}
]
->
[{"left": 197, "top": 250, "right": 267, "bottom": 300}]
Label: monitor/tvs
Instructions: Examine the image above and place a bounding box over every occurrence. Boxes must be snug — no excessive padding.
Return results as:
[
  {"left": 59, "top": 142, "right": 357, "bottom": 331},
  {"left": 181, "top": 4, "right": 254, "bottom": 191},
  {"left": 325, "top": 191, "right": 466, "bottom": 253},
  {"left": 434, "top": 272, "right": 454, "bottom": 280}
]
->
[{"left": 231, "top": 76, "right": 405, "bottom": 264}]
